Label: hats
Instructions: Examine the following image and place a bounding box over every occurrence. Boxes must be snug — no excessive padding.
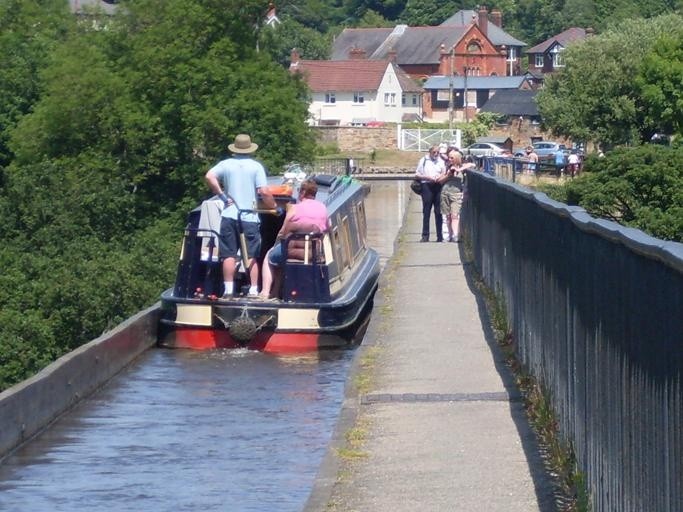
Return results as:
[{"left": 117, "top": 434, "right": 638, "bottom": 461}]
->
[{"left": 227, "top": 134, "right": 259, "bottom": 155}]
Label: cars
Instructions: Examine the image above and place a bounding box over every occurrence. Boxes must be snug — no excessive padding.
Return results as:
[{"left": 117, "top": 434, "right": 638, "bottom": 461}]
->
[
  {"left": 347, "top": 122, "right": 385, "bottom": 128},
  {"left": 458, "top": 142, "right": 512, "bottom": 157}
]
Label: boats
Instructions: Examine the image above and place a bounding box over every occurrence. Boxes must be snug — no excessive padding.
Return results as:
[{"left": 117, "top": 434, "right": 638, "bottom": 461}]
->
[{"left": 156, "top": 174, "right": 382, "bottom": 351}]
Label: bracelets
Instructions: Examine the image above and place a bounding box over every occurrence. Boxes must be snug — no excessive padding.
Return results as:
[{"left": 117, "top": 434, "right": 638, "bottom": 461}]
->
[{"left": 219, "top": 193, "right": 229, "bottom": 202}]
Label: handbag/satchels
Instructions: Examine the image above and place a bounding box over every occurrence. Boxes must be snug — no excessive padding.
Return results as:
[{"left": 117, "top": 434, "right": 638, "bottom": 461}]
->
[{"left": 410, "top": 179, "right": 423, "bottom": 194}]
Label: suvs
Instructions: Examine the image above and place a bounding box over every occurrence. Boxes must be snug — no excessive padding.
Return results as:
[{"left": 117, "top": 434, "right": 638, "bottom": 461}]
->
[{"left": 514, "top": 140, "right": 566, "bottom": 157}]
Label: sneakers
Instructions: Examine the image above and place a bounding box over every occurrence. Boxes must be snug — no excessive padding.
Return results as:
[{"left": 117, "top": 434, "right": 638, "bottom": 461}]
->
[
  {"left": 222, "top": 292, "right": 239, "bottom": 299},
  {"left": 420, "top": 236, "right": 428, "bottom": 242}
]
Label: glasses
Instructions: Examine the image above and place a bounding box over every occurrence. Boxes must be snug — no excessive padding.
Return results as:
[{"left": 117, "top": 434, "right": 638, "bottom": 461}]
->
[{"left": 448, "top": 156, "right": 454, "bottom": 160}]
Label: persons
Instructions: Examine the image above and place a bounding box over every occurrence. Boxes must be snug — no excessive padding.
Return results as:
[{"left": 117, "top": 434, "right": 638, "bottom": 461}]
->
[
  {"left": 204, "top": 134, "right": 277, "bottom": 299},
  {"left": 260, "top": 179, "right": 330, "bottom": 298},
  {"left": 411, "top": 143, "right": 476, "bottom": 242},
  {"left": 523, "top": 145, "right": 581, "bottom": 176}
]
